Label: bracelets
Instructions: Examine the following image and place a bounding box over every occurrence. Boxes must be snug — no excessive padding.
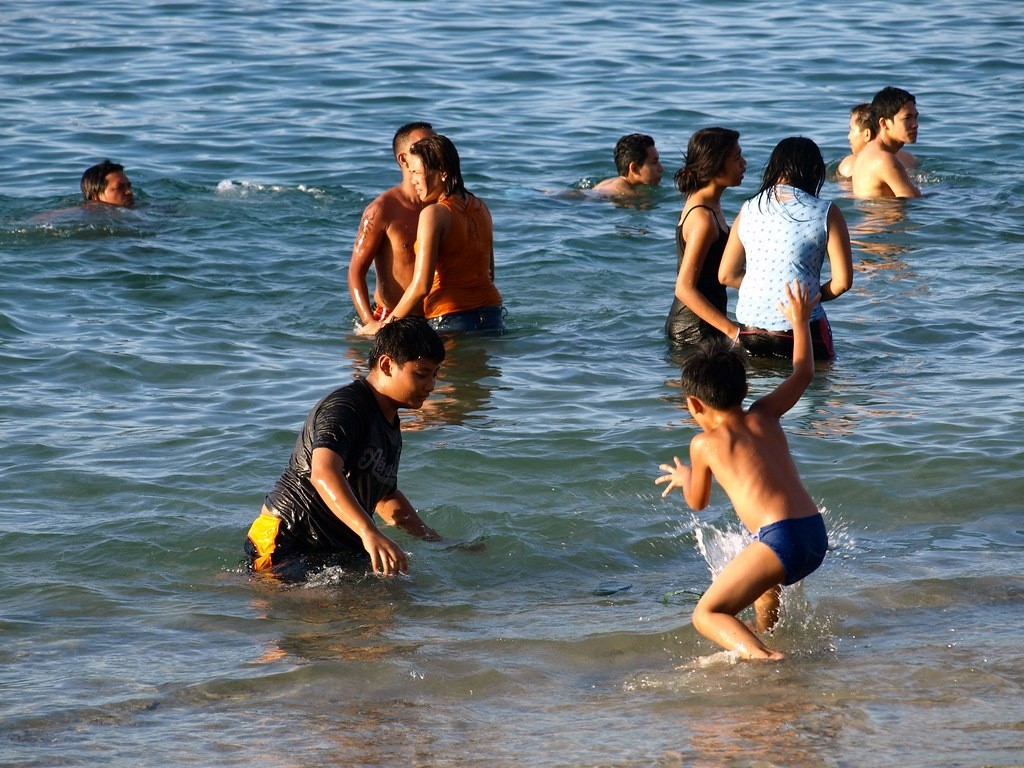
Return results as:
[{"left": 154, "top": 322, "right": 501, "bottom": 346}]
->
[{"left": 729, "top": 321, "right": 741, "bottom": 352}]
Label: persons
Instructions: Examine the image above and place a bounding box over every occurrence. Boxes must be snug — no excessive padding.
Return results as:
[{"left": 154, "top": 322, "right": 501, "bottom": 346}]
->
[
  {"left": 347, "top": 121, "right": 507, "bottom": 339},
  {"left": 243, "top": 314, "right": 447, "bottom": 586},
  {"left": 665, "top": 126, "right": 740, "bottom": 363},
  {"left": 653, "top": 278, "right": 828, "bottom": 661},
  {"left": 835, "top": 86, "right": 923, "bottom": 198},
  {"left": 80, "top": 159, "right": 135, "bottom": 207},
  {"left": 718, "top": 135, "right": 854, "bottom": 361},
  {"left": 592, "top": 133, "right": 664, "bottom": 197}
]
